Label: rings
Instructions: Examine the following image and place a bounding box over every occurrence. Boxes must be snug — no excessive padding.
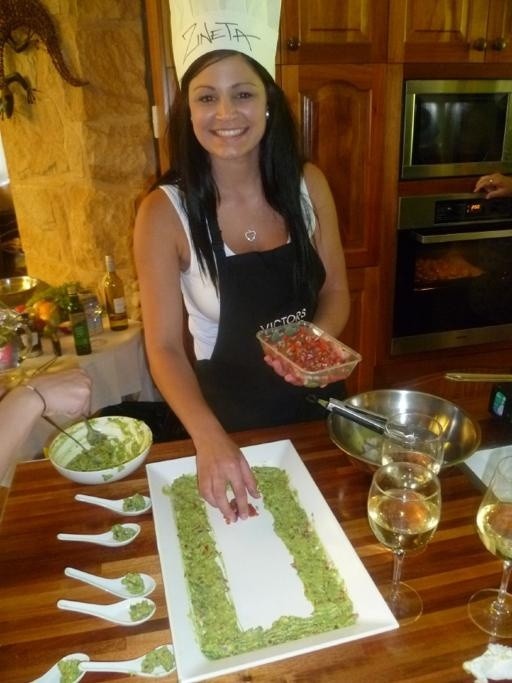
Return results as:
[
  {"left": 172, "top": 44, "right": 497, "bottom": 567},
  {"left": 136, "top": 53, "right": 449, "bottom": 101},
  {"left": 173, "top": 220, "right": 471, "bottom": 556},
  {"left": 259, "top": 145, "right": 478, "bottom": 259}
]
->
[{"left": 489, "top": 177, "right": 493, "bottom": 185}]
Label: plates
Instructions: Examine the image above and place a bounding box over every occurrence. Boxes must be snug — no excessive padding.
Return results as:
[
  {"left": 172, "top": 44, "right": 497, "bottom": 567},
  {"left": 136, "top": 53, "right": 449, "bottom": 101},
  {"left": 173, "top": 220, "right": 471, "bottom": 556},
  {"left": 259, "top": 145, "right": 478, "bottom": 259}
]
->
[
  {"left": 142, "top": 437, "right": 402, "bottom": 681},
  {"left": 462, "top": 444, "right": 512, "bottom": 502}
]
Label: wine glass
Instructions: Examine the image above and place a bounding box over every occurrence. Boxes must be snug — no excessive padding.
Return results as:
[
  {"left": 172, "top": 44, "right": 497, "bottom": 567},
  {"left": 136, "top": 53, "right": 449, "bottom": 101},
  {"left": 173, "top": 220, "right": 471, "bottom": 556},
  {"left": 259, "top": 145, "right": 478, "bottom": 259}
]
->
[
  {"left": 363, "top": 412, "right": 446, "bottom": 630},
  {"left": 465, "top": 453, "right": 512, "bottom": 642}
]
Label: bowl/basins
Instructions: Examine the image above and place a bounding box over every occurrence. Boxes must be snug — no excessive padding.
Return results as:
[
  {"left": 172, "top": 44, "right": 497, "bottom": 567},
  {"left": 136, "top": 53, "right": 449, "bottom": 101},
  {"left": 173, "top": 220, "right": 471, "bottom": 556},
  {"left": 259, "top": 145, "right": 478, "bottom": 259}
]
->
[
  {"left": 322, "top": 388, "right": 483, "bottom": 485},
  {"left": 47, "top": 414, "right": 155, "bottom": 487},
  {"left": 1, "top": 274, "right": 41, "bottom": 307}
]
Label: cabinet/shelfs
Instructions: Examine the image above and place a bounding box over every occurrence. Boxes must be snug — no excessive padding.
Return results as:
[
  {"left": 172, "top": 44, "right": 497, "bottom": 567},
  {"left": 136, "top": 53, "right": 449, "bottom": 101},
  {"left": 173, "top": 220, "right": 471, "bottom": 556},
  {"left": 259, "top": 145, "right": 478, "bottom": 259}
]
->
[
  {"left": 144, "top": 0, "right": 389, "bottom": 65},
  {"left": 388, "top": 0, "right": 512, "bottom": 64},
  {"left": 151, "top": 65, "right": 404, "bottom": 395}
]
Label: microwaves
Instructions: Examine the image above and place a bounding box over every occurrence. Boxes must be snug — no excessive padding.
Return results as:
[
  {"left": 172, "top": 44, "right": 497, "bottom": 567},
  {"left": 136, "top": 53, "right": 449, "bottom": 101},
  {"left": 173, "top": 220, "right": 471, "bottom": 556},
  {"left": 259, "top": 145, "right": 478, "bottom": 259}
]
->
[{"left": 399, "top": 76, "right": 512, "bottom": 180}]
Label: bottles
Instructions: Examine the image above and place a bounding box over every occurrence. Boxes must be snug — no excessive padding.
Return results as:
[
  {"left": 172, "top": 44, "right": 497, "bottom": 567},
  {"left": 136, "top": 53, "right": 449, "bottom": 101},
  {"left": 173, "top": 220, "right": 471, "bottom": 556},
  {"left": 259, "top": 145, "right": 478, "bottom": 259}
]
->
[
  {"left": 65, "top": 284, "right": 94, "bottom": 355},
  {"left": 14, "top": 311, "right": 43, "bottom": 359},
  {"left": 102, "top": 253, "right": 130, "bottom": 332}
]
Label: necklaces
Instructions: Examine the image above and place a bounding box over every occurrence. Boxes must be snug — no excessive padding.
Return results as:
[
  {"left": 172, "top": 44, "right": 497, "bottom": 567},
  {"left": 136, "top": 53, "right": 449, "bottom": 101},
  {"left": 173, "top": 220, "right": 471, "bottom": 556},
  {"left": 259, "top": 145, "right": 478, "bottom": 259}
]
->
[{"left": 243, "top": 226, "right": 257, "bottom": 242}]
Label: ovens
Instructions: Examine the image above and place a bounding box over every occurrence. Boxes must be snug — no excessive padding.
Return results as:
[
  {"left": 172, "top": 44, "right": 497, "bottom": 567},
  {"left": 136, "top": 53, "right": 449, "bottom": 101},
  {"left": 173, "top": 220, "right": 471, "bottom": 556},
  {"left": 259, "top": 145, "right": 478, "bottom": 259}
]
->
[{"left": 389, "top": 189, "right": 512, "bottom": 359}]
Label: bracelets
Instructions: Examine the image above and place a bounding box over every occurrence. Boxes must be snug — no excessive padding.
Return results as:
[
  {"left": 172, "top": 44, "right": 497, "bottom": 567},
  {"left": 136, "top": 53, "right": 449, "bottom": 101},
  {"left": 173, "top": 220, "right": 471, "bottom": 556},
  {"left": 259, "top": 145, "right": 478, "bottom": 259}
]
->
[{"left": 24, "top": 382, "right": 47, "bottom": 419}]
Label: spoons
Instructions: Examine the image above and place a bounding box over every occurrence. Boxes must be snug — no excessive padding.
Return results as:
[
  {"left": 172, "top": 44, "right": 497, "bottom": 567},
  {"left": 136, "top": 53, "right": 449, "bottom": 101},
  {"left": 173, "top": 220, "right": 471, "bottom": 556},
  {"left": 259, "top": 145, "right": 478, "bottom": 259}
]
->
[
  {"left": 27, "top": 652, "right": 91, "bottom": 683},
  {"left": 74, "top": 493, "right": 153, "bottom": 518},
  {"left": 55, "top": 522, "right": 142, "bottom": 549},
  {"left": 56, "top": 596, "right": 157, "bottom": 629},
  {"left": 80, "top": 414, "right": 103, "bottom": 446},
  {"left": 63, "top": 566, "right": 157, "bottom": 600},
  {"left": 77, "top": 642, "right": 178, "bottom": 679}
]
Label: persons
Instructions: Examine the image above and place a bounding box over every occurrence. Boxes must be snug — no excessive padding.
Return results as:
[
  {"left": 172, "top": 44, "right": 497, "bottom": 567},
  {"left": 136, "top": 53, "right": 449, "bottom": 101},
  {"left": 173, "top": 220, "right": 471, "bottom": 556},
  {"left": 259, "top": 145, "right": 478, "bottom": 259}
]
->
[
  {"left": 473, "top": 171, "right": 512, "bottom": 203},
  {"left": 1, "top": 357, "right": 94, "bottom": 486},
  {"left": 130, "top": 47, "right": 353, "bottom": 524}
]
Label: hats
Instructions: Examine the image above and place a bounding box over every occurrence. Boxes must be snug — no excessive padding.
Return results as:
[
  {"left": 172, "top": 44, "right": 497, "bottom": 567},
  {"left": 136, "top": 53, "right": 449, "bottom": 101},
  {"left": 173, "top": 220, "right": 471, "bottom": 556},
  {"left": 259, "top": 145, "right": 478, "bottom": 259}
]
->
[{"left": 168, "top": 2, "right": 283, "bottom": 90}]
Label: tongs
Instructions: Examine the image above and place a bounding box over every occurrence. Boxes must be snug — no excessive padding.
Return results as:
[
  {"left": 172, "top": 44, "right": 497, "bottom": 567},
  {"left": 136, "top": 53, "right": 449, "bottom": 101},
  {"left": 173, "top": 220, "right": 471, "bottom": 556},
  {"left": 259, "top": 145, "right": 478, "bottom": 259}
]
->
[{"left": 305, "top": 387, "right": 439, "bottom": 455}]
